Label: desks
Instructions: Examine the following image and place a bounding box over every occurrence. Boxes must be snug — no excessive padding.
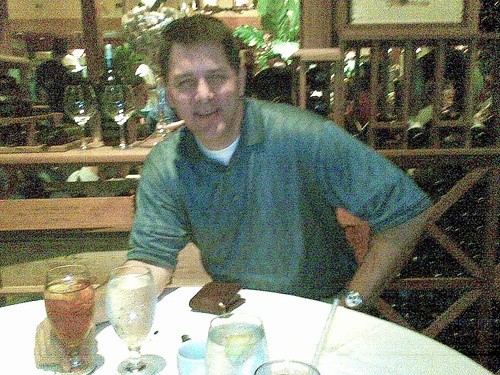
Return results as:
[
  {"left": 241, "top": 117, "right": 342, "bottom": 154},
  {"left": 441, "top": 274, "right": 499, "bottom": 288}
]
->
[
  {"left": 0, "top": 145, "right": 152, "bottom": 164},
  {"left": 0, "top": 287, "right": 495, "bottom": 375}
]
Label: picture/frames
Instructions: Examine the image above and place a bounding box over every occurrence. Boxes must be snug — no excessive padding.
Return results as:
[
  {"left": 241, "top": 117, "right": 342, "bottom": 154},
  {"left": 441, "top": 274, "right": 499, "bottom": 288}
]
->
[{"left": 335, "top": 0, "right": 479, "bottom": 40}]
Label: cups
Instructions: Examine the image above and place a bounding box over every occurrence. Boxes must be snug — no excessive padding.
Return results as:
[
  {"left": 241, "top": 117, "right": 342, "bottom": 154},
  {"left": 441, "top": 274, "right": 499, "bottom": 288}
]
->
[
  {"left": 254, "top": 359, "right": 320, "bottom": 375},
  {"left": 177, "top": 341, "right": 207, "bottom": 375},
  {"left": 205, "top": 313, "right": 271, "bottom": 375}
]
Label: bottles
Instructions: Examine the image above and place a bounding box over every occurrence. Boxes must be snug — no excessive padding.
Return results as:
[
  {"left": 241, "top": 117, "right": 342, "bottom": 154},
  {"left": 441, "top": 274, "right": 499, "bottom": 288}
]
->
[{"left": 99, "top": 44, "right": 128, "bottom": 146}]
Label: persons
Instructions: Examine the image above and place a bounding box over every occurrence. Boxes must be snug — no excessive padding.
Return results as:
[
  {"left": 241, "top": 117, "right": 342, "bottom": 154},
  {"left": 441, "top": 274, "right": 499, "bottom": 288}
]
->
[
  {"left": 33, "top": 13, "right": 433, "bottom": 373},
  {"left": 0, "top": 33, "right": 499, "bottom": 158}
]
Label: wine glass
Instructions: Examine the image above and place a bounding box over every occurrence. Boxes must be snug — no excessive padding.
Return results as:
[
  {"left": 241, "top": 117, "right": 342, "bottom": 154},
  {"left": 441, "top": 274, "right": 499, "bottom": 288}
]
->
[
  {"left": 44, "top": 263, "right": 97, "bottom": 375},
  {"left": 62, "top": 84, "right": 98, "bottom": 149},
  {"left": 101, "top": 84, "right": 136, "bottom": 149},
  {"left": 104, "top": 265, "right": 159, "bottom": 375}
]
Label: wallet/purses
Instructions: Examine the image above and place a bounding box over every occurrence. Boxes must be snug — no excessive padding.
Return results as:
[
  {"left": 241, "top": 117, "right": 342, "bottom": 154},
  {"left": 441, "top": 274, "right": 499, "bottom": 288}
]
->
[{"left": 189, "top": 281, "right": 246, "bottom": 315}]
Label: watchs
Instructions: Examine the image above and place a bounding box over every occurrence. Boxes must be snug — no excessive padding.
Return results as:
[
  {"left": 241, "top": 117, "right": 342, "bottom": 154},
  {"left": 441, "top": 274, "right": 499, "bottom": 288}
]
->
[{"left": 337, "top": 288, "right": 373, "bottom": 313}]
